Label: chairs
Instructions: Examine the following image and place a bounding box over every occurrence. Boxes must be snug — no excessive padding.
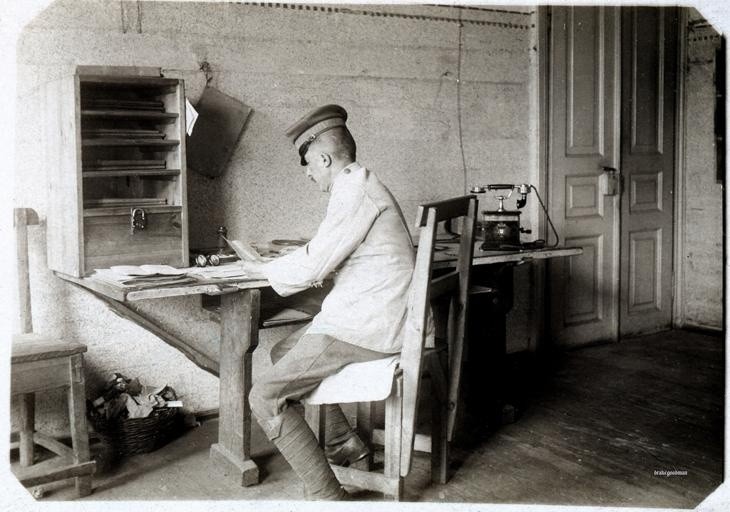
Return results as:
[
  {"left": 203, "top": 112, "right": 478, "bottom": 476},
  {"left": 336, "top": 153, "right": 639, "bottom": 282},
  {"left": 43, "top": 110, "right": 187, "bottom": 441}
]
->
[
  {"left": 11, "top": 207, "right": 96, "bottom": 503},
  {"left": 304, "top": 195, "right": 479, "bottom": 501}
]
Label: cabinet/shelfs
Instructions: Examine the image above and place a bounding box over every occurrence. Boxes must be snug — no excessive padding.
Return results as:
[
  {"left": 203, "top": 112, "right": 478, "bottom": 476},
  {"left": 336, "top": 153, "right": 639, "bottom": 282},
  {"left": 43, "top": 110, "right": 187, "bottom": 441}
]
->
[{"left": 43, "top": 70, "right": 191, "bottom": 277}]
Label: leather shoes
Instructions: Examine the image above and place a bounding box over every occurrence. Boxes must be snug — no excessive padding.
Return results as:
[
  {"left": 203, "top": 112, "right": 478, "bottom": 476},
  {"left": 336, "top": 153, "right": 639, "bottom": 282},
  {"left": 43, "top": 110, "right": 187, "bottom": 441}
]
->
[{"left": 324, "top": 434, "right": 370, "bottom": 467}]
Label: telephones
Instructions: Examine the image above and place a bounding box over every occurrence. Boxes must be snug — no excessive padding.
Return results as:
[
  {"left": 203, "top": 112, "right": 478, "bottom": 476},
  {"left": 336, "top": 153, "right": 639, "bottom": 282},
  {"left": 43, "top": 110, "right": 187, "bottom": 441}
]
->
[{"left": 470, "top": 184, "right": 530, "bottom": 251}]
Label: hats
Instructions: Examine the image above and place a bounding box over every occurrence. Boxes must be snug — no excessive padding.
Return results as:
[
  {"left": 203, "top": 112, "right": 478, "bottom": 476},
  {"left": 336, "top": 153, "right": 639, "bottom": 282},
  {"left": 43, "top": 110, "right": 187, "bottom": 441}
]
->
[{"left": 283, "top": 103, "right": 348, "bottom": 166}]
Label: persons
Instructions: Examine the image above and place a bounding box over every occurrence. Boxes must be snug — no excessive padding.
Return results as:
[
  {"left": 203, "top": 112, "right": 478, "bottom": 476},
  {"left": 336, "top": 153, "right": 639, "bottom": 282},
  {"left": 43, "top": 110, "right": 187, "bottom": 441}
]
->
[{"left": 234, "top": 103, "right": 442, "bottom": 501}]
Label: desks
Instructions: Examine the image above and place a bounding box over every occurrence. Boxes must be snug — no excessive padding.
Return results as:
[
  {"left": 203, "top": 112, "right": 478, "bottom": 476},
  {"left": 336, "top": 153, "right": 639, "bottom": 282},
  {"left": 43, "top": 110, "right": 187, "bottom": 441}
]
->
[{"left": 49, "top": 244, "right": 585, "bottom": 489}]
